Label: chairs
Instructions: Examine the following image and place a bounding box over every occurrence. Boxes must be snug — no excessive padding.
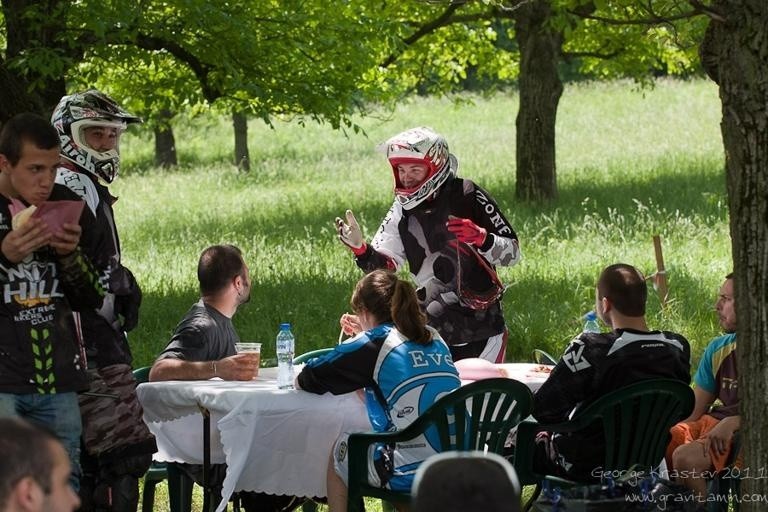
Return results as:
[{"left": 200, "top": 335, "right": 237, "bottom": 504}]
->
[
  {"left": 514, "top": 379, "right": 696, "bottom": 511},
  {"left": 410, "top": 450, "right": 521, "bottom": 512},
  {"left": 132, "top": 366, "right": 227, "bottom": 512},
  {"left": 290, "top": 348, "right": 335, "bottom": 364},
  {"left": 706, "top": 432, "right": 746, "bottom": 512},
  {"left": 347, "top": 377, "right": 534, "bottom": 512}
]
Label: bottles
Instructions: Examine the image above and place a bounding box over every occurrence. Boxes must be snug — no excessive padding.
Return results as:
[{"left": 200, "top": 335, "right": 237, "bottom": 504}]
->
[
  {"left": 276, "top": 323, "right": 296, "bottom": 390},
  {"left": 581, "top": 314, "right": 601, "bottom": 334}
]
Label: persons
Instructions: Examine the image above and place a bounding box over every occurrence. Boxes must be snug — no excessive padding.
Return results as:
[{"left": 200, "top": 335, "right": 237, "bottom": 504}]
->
[
  {"left": 148, "top": 243, "right": 259, "bottom": 492},
  {"left": 51, "top": 89, "right": 159, "bottom": 511},
  {"left": 662, "top": 272, "right": 742, "bottom": 507},
  {"left": 531, "top": 264, "right": 693, "bottom": 477},
  {"left": 332, "top": 122, "right": 524, "bottom": 366},
  {"left": 1, "top": 415, "right": 81, "bottom": 511},
  {"left": 0, "top": 110, "right": 106, "bottom": 495},
  {"left": 294, "top": 266, "right": 471, "bottom": 512}
]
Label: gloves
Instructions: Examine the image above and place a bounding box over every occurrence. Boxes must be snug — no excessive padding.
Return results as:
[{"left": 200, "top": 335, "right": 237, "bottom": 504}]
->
[
  {"left": 335, "top": 210, "right": 366, "bottom": 256},
  {"left": 445, "top": 214, "right": 486, "bottom": 247}
]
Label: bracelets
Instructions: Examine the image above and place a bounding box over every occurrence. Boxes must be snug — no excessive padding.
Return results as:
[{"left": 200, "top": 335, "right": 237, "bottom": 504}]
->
[{"left": 207, "top": 360, "right": 217, "bottom": 379}]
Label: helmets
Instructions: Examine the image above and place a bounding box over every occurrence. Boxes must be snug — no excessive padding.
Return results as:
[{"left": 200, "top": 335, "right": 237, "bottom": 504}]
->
[
  {"left": 50, "top": 90, "right": 143, "bottom": 184},
  {"left": 386, "top": 127, "right": 450, "bottom": 212}
]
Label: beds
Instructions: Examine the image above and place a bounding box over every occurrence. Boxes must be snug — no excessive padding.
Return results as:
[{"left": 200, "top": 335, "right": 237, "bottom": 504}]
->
[{"left": 137, "top": 362, "right": 670, "bottom": 512}]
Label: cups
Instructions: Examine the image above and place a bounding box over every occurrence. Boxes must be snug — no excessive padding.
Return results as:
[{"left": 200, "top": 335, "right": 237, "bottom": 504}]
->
[{"left": 234, "top": 342, "right": 263, "bottom": 378}]
